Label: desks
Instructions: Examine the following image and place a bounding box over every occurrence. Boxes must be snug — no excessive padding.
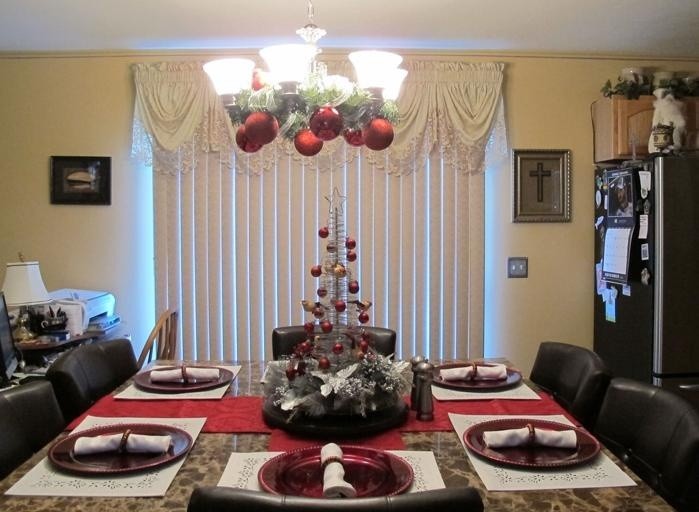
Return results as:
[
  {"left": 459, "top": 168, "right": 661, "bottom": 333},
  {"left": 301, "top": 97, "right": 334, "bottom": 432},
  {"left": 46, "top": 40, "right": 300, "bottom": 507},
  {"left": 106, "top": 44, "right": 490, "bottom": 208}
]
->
[{"left": 13, "top": 328, "right": 107, "bottom": 367}]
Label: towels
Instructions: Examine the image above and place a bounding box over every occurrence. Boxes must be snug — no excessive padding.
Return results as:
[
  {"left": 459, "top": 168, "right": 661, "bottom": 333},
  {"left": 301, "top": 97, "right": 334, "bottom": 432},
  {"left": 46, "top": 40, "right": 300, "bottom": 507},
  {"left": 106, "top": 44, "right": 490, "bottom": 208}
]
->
[
  {"left": 75, "top": 429, "right": 172, "bottom": 456},
  {"left": 151, "top": 368, "right": 220, "bottom": 383},
  {"left": 483, "top": 427, "right": 578, "bottom": 451},
  {"left": 318, "top": 441, "right": 357, "bottom": 497},
  {"left": 441, "top": 364, "right": 509, "bottom": 379}
]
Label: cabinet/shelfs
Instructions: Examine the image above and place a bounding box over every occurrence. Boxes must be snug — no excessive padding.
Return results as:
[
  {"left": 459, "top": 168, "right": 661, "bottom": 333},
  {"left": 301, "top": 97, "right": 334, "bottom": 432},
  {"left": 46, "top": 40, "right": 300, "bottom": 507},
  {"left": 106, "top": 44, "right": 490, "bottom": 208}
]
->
[{"left": 590, "top": 91, "right": 699, "bottom": 161}]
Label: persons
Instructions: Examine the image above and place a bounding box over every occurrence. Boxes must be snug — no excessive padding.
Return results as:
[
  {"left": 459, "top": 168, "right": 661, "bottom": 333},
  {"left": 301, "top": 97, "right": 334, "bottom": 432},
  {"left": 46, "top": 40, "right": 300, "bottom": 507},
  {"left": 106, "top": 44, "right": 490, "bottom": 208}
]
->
[{"left": 615, "top": 175, "right": 633, "bottom": 216}]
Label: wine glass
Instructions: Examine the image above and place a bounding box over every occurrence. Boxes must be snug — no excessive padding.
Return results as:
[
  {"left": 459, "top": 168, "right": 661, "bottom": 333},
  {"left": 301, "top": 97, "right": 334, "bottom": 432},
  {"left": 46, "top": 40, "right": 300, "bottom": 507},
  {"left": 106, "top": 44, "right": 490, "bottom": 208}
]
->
[{"left": 652, "top": 125, "right": 674, "bottom": 156}]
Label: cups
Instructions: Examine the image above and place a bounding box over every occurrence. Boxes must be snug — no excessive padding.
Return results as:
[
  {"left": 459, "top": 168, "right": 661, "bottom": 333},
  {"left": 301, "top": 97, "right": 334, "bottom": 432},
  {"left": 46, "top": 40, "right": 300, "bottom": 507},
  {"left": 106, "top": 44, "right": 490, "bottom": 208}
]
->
[{"left": 42, "top": 311, "right": 65, "bottom": 328}]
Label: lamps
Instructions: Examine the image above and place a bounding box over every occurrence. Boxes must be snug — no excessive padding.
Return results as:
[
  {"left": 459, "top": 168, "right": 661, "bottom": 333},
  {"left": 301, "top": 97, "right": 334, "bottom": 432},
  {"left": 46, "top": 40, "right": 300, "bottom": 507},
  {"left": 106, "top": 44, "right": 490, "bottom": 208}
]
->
[
  {"left": 2, "top": 261, "right": 51, "bottom": 339},
  {"left": 203, "top": 1, "right": 409, "bottom": 157}
]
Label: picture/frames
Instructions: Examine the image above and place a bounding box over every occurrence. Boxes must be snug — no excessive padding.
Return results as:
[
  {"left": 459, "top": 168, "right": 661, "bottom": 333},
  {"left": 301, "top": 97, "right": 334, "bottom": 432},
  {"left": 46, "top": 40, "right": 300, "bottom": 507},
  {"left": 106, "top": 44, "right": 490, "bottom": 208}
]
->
[
  {"left": 50, "top": 155, "right": 112, "bottom": 206},
  {"left": 511, "top": 148, "right": 570, "bottom": 223}
]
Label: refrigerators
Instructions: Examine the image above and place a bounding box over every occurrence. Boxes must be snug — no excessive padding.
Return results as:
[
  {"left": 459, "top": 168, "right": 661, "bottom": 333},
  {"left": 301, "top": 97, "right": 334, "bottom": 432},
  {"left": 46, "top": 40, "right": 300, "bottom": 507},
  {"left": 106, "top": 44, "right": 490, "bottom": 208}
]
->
[{"left": 594, "top": 157, "right": 698, "bottom": 407}]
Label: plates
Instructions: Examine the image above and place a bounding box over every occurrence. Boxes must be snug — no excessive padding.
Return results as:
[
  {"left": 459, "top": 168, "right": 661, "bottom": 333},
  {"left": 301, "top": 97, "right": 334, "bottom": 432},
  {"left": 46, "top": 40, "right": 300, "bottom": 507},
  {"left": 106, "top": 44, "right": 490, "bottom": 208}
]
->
[
  {"left": 464, "top": 419, "right": 600, "bottom": 466},
  {"left": 433, "top": 362, "right": 522, "bottom": 388},
  {"left": 258, "top": 445, "right": 414, "bottom": 498},
  {"left": 135, "top": 365, "right": 233, "bottom": 390},
  {"left": 48, "top": 423, "right": 193, "bottom": 473}
]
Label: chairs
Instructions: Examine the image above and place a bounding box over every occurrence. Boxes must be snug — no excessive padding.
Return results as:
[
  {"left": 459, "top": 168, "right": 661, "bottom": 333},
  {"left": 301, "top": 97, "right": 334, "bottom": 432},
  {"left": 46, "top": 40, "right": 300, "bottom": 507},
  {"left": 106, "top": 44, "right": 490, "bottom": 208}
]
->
[
  {"left": 528, "top": 340, "right": 610, "bottom": 418},
  {"left": 271, "top": 325, "right": 397, "bottom": 365},
  {"left": 136, "top": 307, "right": 178, "bottom": 370},
  {"left": 46, "top": 335, "right": 139, "bottom": 419},
  {"left": 596, "top": 377, "right": 699, "bottom": 499},
  {"left": 0, "top": 381, "right": 66, "bottom": 489},
  {"left": 186, "top": 485, "right": 485, "bottom": 512}
]
